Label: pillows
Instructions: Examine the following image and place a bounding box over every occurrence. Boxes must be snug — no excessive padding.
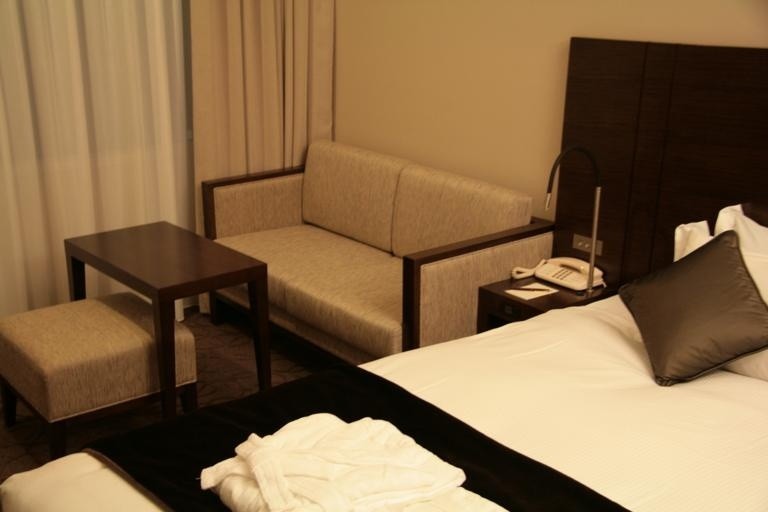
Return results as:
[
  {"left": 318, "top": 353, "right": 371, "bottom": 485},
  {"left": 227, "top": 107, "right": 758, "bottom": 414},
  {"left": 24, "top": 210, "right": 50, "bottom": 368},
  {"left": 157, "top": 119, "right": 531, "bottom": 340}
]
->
[{"left": 609, "top": 204, "right": 768, "bottom": 392}]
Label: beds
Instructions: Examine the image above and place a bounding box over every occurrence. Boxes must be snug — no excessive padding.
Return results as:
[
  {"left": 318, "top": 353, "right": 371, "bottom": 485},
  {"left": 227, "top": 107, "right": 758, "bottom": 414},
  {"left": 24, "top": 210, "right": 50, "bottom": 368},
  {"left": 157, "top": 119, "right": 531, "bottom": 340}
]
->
[{"left": 0, "top": 34, "right": 768, "bottom": 512}]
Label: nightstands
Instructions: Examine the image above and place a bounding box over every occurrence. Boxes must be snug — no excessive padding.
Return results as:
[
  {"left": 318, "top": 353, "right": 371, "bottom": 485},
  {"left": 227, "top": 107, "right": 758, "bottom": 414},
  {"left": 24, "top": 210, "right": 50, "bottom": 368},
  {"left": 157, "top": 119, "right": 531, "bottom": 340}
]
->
[{"left": 477, "top": 275, "right": 607, "bottom": 336}]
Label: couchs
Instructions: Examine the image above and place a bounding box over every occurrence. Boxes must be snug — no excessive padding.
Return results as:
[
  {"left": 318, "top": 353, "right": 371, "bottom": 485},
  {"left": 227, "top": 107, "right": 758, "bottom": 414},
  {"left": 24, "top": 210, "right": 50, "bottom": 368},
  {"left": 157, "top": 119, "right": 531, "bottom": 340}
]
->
[{"left": 199, "top": 140, "right": 558, "bottom": 355}]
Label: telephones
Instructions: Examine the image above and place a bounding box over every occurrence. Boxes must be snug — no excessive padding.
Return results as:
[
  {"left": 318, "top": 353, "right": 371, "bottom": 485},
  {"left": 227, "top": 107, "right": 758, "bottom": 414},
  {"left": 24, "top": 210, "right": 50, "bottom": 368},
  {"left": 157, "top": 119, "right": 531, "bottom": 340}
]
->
[{"left": 534, "top": 257, "right": 603, "bottom": 291}]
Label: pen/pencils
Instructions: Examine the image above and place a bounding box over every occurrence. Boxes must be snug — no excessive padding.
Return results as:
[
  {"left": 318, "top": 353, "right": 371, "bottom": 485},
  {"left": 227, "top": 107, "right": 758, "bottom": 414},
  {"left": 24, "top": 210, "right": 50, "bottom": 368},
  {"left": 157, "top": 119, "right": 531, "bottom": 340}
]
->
[{"left": 510, "top": 287, "right": 550, "bottom": 291}]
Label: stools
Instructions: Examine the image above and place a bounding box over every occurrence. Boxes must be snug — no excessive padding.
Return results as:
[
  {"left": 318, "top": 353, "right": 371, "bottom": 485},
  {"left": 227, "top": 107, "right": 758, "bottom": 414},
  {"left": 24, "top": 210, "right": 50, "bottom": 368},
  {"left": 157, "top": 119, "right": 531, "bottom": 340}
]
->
[{"left": 0, "top": 292, "right": 200, "bottom": 440}]
